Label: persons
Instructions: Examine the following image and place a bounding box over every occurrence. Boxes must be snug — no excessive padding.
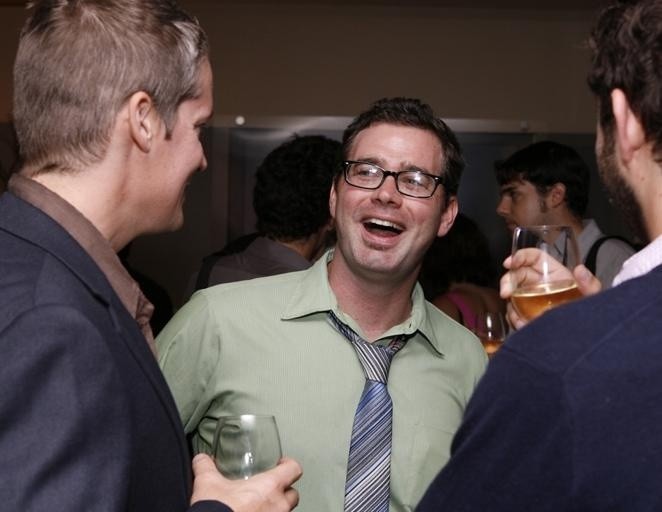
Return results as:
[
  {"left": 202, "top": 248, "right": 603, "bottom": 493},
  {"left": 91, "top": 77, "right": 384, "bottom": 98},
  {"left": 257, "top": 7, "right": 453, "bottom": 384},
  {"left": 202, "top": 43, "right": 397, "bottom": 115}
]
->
[
  {"left": 0, "top": 0, "right": 302, "bottom": 512},
  {"left": 415, "top": 0, "right": 662, "bottom": 511},
  {"left": 195, "top": 135, "right": 344, "bottom": 290},
  {"left": 155, "top": 98, "right": 490, "bottom": 511},
  {"left": 418, "top": 213, "right": 509, "bottom": 361},
  {"left": 493, "top": 140, "right": 636, "bottom": 291}
]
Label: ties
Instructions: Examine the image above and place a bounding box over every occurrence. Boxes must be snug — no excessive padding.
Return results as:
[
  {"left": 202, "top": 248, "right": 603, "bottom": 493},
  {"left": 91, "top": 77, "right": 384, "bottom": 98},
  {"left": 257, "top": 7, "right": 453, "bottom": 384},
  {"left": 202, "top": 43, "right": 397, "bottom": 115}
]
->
[{"left": 329, "top": 312, "right": 409, "bottom": 511}]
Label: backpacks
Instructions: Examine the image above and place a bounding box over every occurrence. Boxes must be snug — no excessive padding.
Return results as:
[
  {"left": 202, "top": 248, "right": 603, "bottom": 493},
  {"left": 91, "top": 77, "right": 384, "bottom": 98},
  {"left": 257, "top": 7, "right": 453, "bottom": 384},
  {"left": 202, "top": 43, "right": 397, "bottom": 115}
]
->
[{"left": 584, "top": 235, "right": 645, "bottom": 276}]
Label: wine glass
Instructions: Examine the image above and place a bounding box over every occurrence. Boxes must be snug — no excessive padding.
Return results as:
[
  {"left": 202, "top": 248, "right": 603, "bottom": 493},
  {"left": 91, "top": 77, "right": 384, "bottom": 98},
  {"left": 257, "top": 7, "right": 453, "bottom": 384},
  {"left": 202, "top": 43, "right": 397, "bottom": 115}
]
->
[
  {"left": 509, "top": 222, "right": 586, "bottom": 320},
  {"left": 470, "top": 309, "right": 507, "bottom": 364},
  {"left": 210, "top": 416, "right": 283, "bottom": 482}
]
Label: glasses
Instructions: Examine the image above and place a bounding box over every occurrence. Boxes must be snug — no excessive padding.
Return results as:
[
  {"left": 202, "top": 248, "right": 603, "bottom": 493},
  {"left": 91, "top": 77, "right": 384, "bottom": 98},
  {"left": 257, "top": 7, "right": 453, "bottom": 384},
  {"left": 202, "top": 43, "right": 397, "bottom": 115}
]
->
[{"left": 340, "top": 161, "right": 444, "bottom": 199}]
